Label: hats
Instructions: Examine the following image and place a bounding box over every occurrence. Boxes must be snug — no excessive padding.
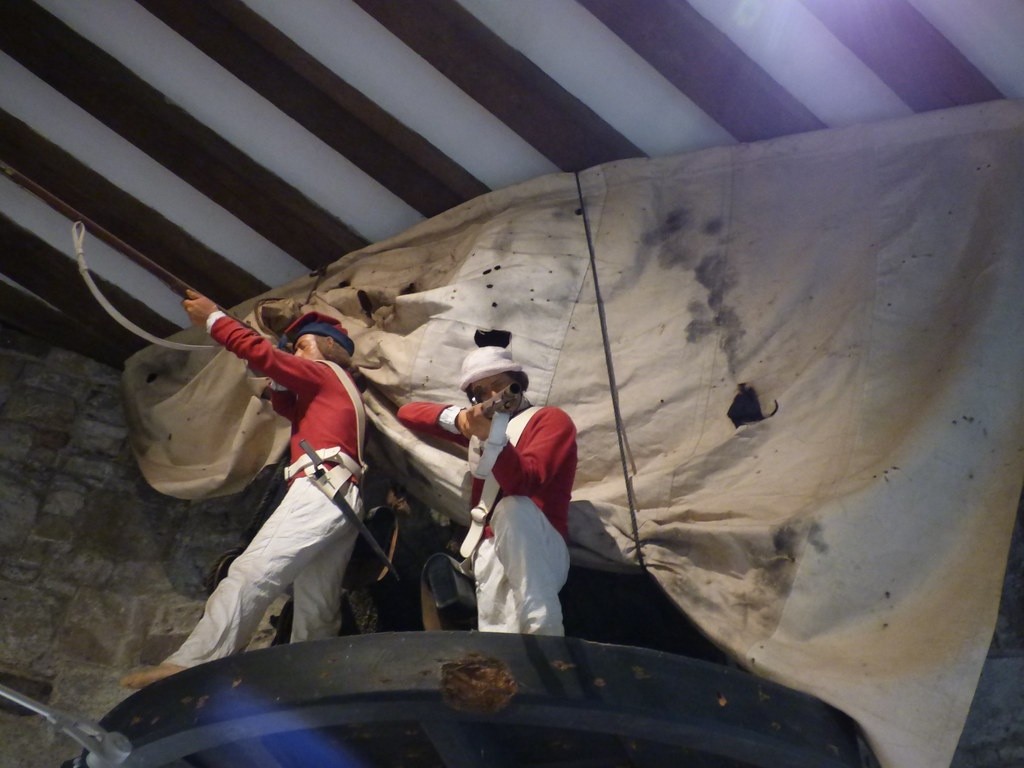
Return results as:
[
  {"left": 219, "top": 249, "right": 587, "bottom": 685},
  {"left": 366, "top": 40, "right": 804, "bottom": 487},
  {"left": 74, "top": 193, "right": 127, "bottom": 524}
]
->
[
  {"left": 460, "top": 344, "right": 522, "bottom": 392},
  {"left": 278, "top": 312, "right": 356, "bottom": 356}
]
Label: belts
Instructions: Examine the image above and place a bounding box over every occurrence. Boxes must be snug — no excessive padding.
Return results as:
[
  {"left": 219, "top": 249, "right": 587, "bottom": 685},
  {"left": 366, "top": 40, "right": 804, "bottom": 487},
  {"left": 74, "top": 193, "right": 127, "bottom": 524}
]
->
[{"left": 285, "top": 446, "right": 363, "bottom": 481}]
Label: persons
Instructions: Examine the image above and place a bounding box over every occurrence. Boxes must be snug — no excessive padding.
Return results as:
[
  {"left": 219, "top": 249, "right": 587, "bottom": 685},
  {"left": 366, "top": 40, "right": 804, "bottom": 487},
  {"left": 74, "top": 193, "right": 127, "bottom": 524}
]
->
[
  {"left": 120, "top": 289, "right": 370, "bottom": 690},
  {"left": 396, "top": 346, "right": 578, "bottom": 637}
]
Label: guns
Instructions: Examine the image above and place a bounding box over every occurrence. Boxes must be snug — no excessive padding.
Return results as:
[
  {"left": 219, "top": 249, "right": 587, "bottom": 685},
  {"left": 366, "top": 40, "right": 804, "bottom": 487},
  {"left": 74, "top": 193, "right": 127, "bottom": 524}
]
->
[
  {"left": 0, "top": 159, "right": 263, "bottom": 337},
  {"left": 468, "top": 382, "right": 521, "bottom": 417}
]
